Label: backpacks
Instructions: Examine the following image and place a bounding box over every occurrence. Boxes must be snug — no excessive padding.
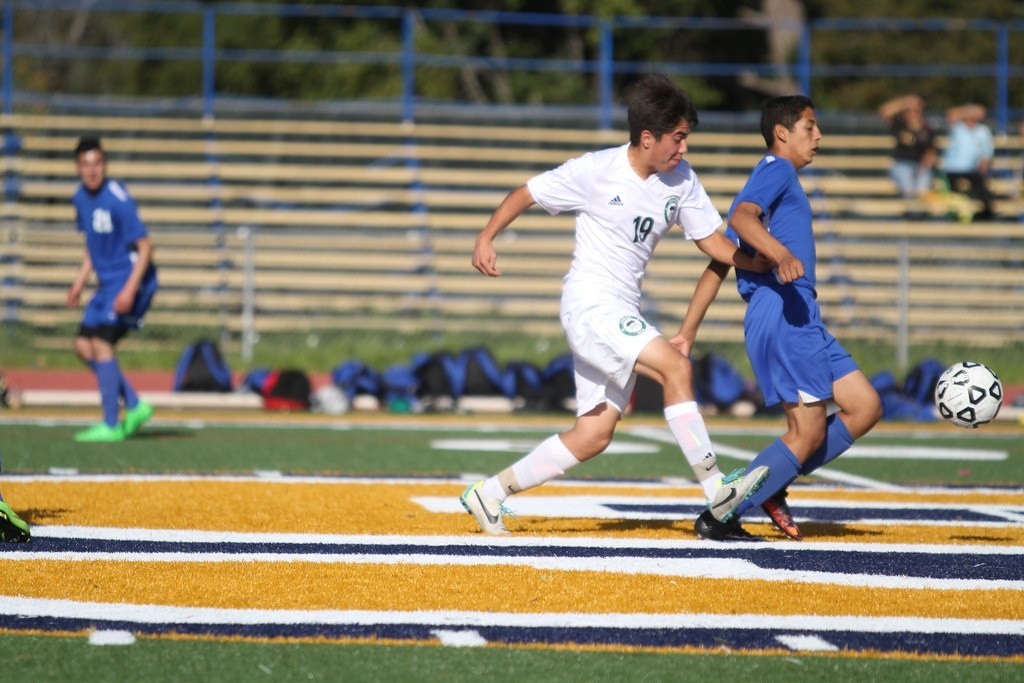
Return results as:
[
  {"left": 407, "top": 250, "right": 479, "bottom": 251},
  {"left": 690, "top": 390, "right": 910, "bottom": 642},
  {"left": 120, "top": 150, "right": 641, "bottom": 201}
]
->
[
  {"left": 173, "top": 340, "right": 232, "bottom": 391},
  {"left": 243, "top": 346, "right": 949, "bottom": 416}
]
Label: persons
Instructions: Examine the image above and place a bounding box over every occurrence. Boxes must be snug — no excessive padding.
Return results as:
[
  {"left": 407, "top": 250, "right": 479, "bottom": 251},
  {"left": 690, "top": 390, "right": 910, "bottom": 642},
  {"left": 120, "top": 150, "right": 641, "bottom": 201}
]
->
[
  {"left": 66, "top": 136, "right": 159, "bottom": 444},
  {"left": 667, "top": 95, "right": 883, "bottom": 542},
  {"left": 460, "top": 74, "right": 777, "bottom": 538},
  {"left": 881, "top": 93, "right": 941, "bottom": 220},
  {"left": 940, "top": 103, "right": 995, "bottom": 227}
]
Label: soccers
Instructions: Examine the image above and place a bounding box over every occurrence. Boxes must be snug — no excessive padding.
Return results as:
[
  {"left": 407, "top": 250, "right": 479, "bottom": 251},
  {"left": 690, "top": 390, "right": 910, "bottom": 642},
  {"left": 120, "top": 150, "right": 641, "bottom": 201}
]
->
[{"left": 935, "top": 360, "right": 1003, "bottom": 429}]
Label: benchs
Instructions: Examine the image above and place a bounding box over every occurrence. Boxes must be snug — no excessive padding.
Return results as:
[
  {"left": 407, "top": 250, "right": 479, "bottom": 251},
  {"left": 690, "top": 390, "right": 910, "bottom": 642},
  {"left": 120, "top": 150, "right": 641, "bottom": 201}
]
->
[{"left": 0, "top": 113, "right": 1023, "bottom": 345}]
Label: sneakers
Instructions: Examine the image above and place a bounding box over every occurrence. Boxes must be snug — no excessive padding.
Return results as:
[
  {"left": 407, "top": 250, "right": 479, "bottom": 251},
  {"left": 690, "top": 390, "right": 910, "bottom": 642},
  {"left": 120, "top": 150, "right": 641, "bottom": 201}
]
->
[
  {"left": 760, "top": 488, "right": 802, "bottom": 542},
  {"left": 74, "top": 421, "right": 127, "bottom": 443},
  {"left": 708, "top": 466, "right": 770, "bottom": 523},
  {"left": 0, "top": 500, "right": 31, "bottom": 543},
  {"left": 694, "top": 515, "right": 763, "bottom": 542},
  {"left": 123, "top": 403, "right": 151, "bottom": 436},
  {"left": 459, "top": 479, "right": 518, "bottom": 535}
]
